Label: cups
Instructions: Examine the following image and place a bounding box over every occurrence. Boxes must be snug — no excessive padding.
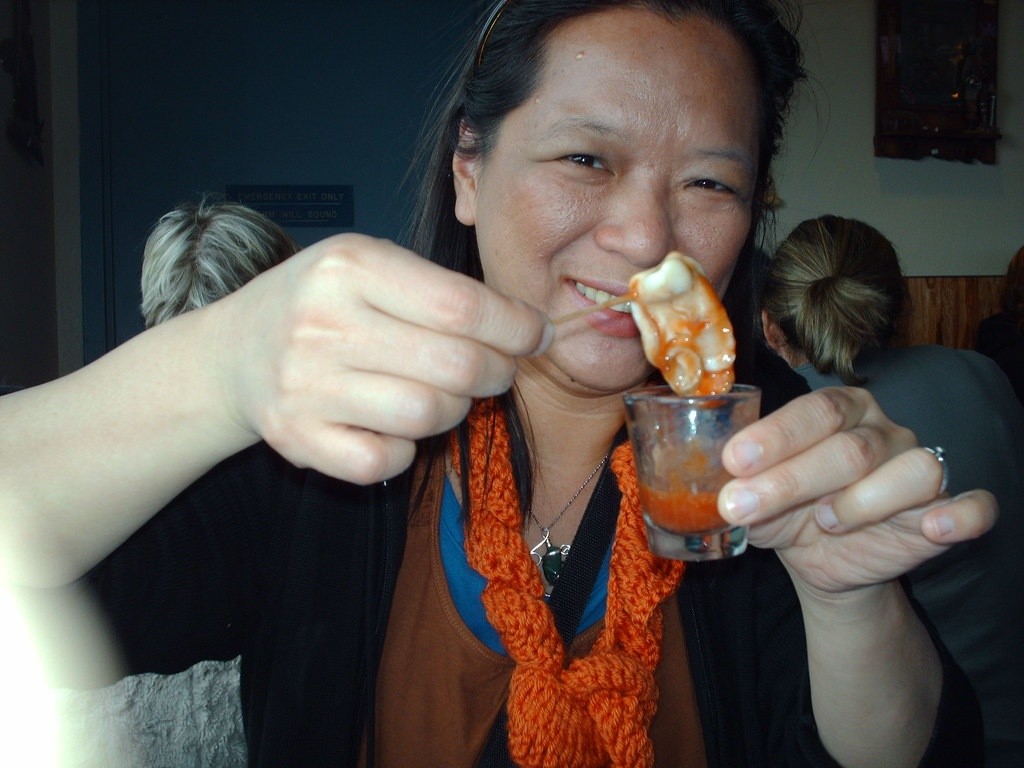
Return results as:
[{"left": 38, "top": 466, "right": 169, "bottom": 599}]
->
[{"left": 622, "top": 377, "right": 764, "bottom": 559}]
